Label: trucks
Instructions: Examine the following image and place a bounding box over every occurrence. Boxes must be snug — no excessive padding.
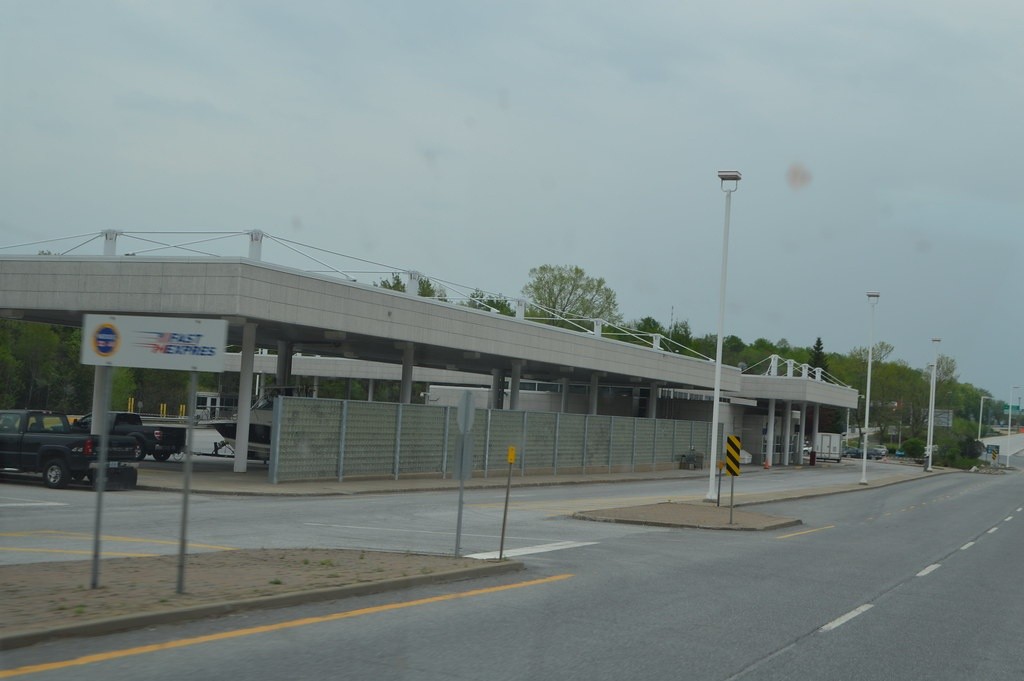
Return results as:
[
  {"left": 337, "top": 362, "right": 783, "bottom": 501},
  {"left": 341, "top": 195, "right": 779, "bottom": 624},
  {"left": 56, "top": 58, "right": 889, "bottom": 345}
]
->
[{"left": 815, "top": 433, "right": 843, "bottom": 463}]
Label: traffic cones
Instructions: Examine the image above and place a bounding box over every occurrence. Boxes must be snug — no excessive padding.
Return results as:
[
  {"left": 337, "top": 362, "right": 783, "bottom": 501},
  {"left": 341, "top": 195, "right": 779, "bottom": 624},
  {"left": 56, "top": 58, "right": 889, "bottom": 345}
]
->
[
  {"left": 884, "top": 456, "right": 886, "bottom": 461},
  {"left": 764, "top": 457, "right": 769, "bottom": 469}
]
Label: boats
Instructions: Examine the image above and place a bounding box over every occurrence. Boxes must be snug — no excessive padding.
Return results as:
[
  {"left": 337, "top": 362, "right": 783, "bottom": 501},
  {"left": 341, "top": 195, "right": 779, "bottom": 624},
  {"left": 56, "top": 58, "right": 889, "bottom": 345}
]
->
[{"left": 197, "top": 385, "right": 314, "bottom": 460}]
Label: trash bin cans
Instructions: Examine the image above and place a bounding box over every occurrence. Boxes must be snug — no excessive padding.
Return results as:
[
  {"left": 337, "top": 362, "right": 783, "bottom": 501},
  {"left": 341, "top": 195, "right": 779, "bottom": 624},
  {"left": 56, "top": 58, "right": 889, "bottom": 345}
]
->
[{"left": 809, "top": 451, "right": 816, "bottom": 466}]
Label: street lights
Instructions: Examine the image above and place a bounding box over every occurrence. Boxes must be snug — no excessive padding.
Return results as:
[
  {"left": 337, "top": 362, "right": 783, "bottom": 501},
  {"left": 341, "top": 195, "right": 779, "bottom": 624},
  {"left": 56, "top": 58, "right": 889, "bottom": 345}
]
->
[
  {"left": 1005, "top": 385, "right": 1020, "bottom": 467},
  {"left": 978, "top": 396, "right": 991, "bottom": 442},
  {"left": 926, "top": 337, "right": 942, "bottom": 472},
  {"left": 704, "top": 171, "right": 743, "bottom": 503},
  {"left": 859, "top": 291, "right": 880, "bottom": 485},
  {"left": 846, "top": 395, "right": 865, "bottom": 446}
]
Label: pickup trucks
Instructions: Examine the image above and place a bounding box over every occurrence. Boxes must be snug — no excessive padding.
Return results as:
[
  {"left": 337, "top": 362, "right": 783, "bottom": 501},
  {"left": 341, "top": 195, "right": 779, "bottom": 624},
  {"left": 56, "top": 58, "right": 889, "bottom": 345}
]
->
[
  {"left": 0, "top": 410, "right": 138, "bottom": 489},
  {"left": 49, "top": 410, "right": 186, "bottom": 461}
]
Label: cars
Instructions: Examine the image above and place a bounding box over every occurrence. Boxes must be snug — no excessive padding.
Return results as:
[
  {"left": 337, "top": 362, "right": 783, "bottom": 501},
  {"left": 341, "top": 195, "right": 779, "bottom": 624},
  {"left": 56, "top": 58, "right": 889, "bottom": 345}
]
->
[
  {"left": 803, "top": 447, "right": 812, "bottom": 456},
  {"left": 875, "top": 445, "right": 888, "bottom": 457},
  {"left": 842, "top": 446, "right": 882, "bottom": 460}
]
